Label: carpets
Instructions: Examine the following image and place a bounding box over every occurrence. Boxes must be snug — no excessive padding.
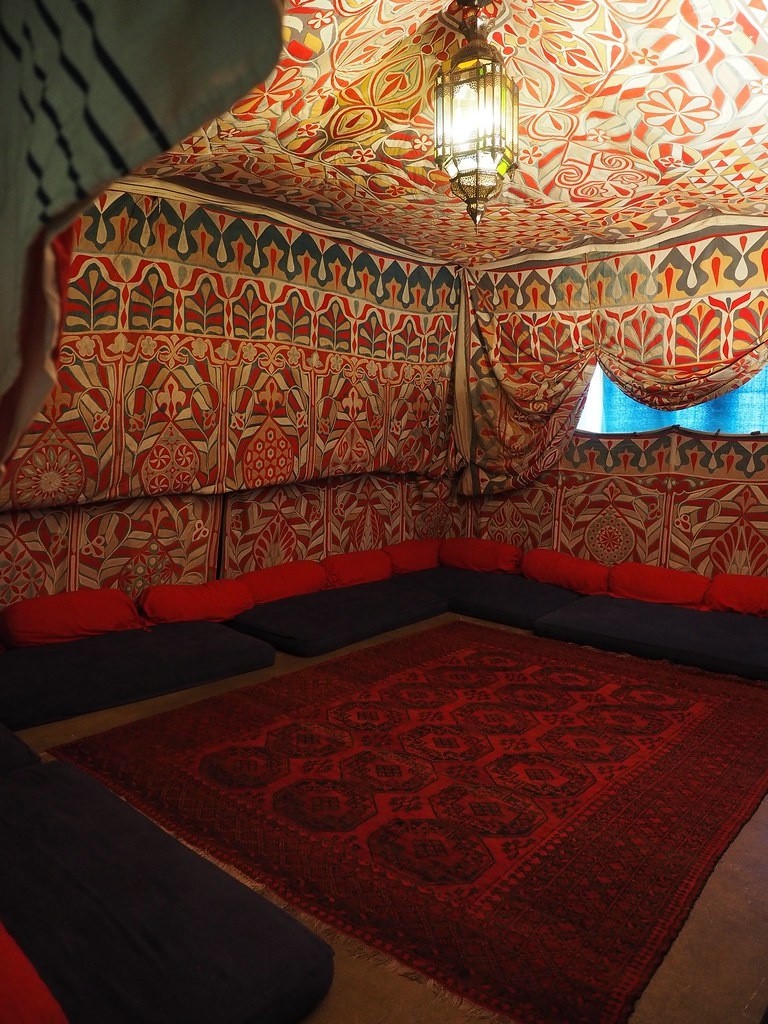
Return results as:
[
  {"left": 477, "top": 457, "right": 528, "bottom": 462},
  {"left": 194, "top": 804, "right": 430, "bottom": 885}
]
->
[{"left": 49, "top": 618, "right": 768, "bottom": 1024}]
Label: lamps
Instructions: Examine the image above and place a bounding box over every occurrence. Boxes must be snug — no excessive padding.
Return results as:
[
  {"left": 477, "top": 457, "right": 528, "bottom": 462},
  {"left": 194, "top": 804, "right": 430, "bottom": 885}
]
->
[{"left": 433, "top": 0, "right": 519, "bottom": 235}]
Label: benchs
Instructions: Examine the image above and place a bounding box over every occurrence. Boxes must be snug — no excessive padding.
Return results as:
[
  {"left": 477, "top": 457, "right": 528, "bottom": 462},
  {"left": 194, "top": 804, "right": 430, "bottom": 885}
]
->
[{"left": 0, "top": 566, "right": 768, "bottom": 1024}]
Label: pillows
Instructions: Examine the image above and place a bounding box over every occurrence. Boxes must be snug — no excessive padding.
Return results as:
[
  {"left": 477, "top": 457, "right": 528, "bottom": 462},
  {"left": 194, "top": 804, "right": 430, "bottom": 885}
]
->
[
  {"left": 443, "top": 537, "right": 524, "bottom": 575},
  {"left": 320, "top": 550, "right": 394, "bottom": 588},
  {"left": 0, "top": 587, "right": 157, "bottom": 648},
  {"left": 138, "top": 578, "right": 255, "bottom": 622},
  {"left": 237, "top": 559, "right": 327, "bottom": 604},
  {"left": 610, "top": 561, "right": 711, "bottom": 613},
  {"left": 521, "top": 548, "right": 612, "bottom": 596},
  {"left": 701, "top": 573, "right": 768, "bottom": 618},
  {"left": 380, "top": 538, "right": 442, "bottom": 574}
]
